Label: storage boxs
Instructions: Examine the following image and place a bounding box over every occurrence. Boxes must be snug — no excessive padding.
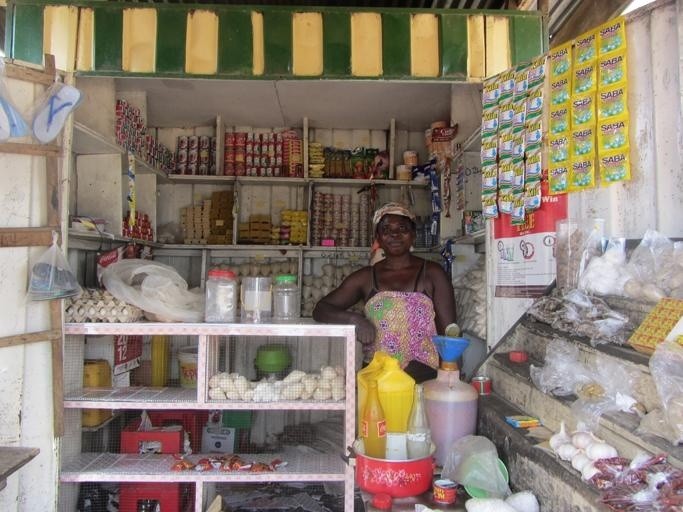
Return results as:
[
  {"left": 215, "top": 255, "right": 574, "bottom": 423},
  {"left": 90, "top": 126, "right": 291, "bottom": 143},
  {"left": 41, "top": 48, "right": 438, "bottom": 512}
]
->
[
  {"left": 83, "top": 360, "right": 112, "bottom": 426},
  {"left": 120, "top": 413, "right": 207, "bottom": 512}
]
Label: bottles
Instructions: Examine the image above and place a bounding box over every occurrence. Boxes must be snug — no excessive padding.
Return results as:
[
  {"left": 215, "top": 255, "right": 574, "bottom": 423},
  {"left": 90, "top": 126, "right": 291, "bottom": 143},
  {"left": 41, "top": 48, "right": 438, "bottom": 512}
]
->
[
  {"left": 364, "top": 380, "right": 387, "bottom": 458},
  {"left": 407, "top": 382, "right": 431, "bottom": 460}
]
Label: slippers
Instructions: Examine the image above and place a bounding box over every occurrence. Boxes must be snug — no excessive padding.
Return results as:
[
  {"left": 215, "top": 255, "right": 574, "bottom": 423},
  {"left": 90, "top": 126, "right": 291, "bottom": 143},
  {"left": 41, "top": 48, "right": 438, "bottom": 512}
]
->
[
  {"left": 0, "top": 103, "right": 10, "bottom": 146},
  {"left": 33, "top": 84, "right": 80, "bottom": 145}
]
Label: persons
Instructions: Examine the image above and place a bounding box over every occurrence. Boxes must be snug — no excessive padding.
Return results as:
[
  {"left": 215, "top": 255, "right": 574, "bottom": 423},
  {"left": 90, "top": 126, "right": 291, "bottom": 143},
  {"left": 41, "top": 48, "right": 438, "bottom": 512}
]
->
[{"left": 312, "top": 205, "right": 457, "bottom": 385}]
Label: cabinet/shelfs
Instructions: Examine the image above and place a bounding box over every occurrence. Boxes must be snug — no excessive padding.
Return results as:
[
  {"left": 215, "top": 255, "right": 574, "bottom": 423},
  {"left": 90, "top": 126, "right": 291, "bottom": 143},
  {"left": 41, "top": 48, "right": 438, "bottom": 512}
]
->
[
  {"left": 167, "top": 114, "right": 432, "bottom": 320},
  {"left": 61, "top": 76, "right": 167, "bottom": 428},
  {"left": 430, "top": 83, "right": 555, "bottom": 382},
  {"left": 467, "top": 239, "right": 683, "bottom": 512},
  {"left": 58, "top": 319, "right": 357, "bottom": 512}
]
